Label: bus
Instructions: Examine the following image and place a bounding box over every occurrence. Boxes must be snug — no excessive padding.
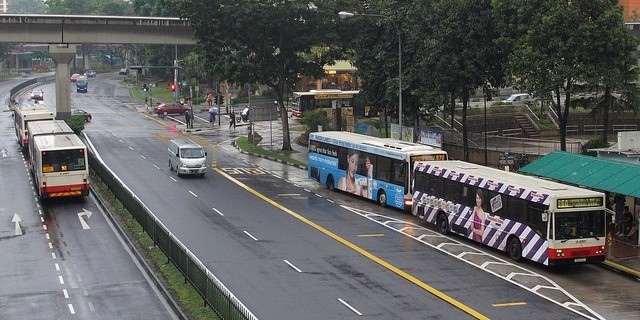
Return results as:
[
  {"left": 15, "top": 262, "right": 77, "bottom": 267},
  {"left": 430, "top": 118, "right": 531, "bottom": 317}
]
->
[
  {"left": 410, "top": 159, "right": 609, "bottom": 269},
  {"left": 292, "top": 90, "right": 384, "bottom": 118},
  {"left": 11, "top": 105, "right": 55, "bottom": 149},
  {"left": 26, "top": 118, "right": 89, "bottom": 200},
  {"left": 307, "top": 130, "right": 449, "bottom": 213}
]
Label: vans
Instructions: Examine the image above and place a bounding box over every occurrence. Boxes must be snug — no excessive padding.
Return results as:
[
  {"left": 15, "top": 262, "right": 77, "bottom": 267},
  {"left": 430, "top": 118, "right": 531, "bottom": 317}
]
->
[{"left": 166, "top": 138, "right": 209, "bottom": 180}]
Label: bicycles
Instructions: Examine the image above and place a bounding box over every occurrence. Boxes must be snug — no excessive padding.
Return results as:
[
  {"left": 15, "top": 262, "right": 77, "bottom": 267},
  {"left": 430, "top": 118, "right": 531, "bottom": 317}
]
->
[{"left": 496, "top": 148, "right": 529, "bottom": 170}]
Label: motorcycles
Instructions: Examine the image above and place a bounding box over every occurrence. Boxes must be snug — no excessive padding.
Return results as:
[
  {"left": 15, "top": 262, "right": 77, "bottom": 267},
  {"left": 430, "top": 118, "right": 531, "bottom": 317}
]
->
[{"left": 236, "top": 112, "right": 250, "bottom": 122}]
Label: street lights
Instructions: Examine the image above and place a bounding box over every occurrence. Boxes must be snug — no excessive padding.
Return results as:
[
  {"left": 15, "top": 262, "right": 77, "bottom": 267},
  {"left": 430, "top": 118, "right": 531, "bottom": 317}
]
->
[{"left": 338, "top": 11, "right": 403, "bottom": 140}]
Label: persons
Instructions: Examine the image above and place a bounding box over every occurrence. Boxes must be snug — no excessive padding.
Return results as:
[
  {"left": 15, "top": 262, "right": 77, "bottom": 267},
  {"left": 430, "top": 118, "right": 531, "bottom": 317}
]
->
[
  {"left": 620, "top": 213, "right": 629, "bottom": 240},
  {"left": 209, "top": 113, "right": 214, "bottom": 126},
  {"left": 628, "top": 209, "right": 640, "bottom": 237},
  {"left": 229, "top": 109, "right": 236, "bottom": 130},
  {"left": 616, "top": 205, "right": 633, "bottom": 236},
  {"left": 207, "top": 92, "right": 213, "bottom": 106},
  {"left": 611, "top": 194, "right": 626, "bottom": 228},
  {"left": 470, "top": 188, "right": 485, "bottom": 243},
  {"left": 337, "top": 149, "right": 363, "bottom": 197},
  {"left": 184, "top": 111, "right": 191, "bottom": 129}
]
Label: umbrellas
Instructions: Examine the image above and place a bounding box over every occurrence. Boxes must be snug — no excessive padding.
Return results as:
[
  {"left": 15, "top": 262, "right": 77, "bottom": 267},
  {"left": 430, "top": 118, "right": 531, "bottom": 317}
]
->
[{"left": 208, "top": 107, "right": 218, "bottom": 116}]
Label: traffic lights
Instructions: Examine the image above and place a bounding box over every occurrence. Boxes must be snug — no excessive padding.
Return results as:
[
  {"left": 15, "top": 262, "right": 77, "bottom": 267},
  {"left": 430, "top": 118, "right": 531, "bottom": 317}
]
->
[
  {"left": 220, "top": 95, "right": 225, "bottom": 104},
  {"left": 214, "top": 95, "right": 217, "bottom": 104}
]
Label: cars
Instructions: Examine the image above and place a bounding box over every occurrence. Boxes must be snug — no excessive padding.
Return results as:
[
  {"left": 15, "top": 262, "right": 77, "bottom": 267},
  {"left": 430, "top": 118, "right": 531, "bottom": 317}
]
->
[
  {"left": 70, "top": 73, "right": 81, "bottom": 82},
  {"left": 119, "top": 68, "right": 129, "bottom": 75},
  {"left": 502, "top": 93, "right": 533, "bottom": 108},
  {"left": 30, "top": 88, "right": 44, "bottom": 100},
  {"left": 86, "top": 70, "right": 97, "bottom": 78},
  {"left": 153, "top": 102, "right": 192, "bottom": 115},
  {"left": 77, "top": 77, "right": 89, "bottom": 92},
  {"left": 72, "top": 109, "right": 92, "bottom": 123}
]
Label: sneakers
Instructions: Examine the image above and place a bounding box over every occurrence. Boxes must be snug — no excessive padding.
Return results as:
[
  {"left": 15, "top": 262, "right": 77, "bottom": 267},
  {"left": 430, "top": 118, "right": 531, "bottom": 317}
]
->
[
  {"left": 618, "top": 233, "right": 624, "bottom": 236},
  {"left": 615, "top": 231, "right": 619, "bottom": 236}
]
